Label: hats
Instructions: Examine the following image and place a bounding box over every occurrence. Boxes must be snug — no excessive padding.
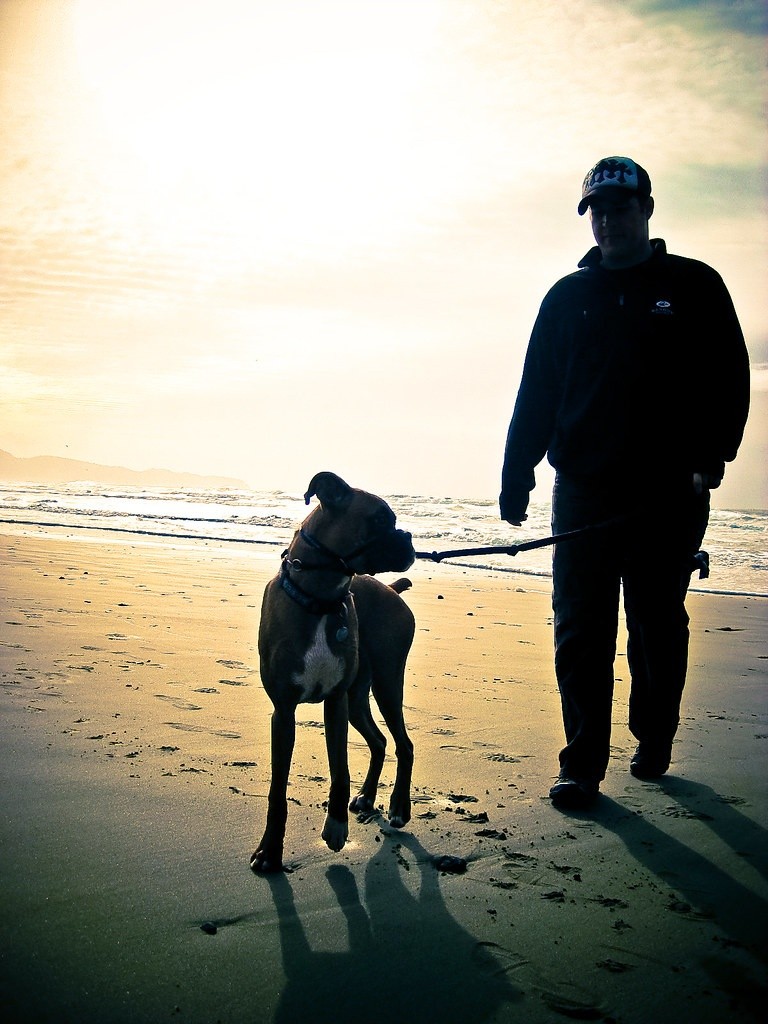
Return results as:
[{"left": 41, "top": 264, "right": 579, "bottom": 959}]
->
[{"left": 578, "top": 156, "right": 651, "bottom": 216}]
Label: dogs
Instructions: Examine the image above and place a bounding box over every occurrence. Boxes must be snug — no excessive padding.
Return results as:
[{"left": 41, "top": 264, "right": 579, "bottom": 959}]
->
[{"left": 248, "top": 471, "right": 417, "bottom": 879}]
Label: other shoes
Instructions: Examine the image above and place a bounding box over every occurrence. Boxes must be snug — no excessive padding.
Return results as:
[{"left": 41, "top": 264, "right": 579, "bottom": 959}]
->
[
  {"left": 630, "top": 717, "right": 680, "bottom": 778},
  {"left": 549, "top": 767, "right": 600, "bottom": 800}
]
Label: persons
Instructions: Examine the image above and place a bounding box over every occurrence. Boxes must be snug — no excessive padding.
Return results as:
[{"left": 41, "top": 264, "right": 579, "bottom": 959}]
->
[{"left": 499, "top": 157, "right": 751, "bottom": 802}]
